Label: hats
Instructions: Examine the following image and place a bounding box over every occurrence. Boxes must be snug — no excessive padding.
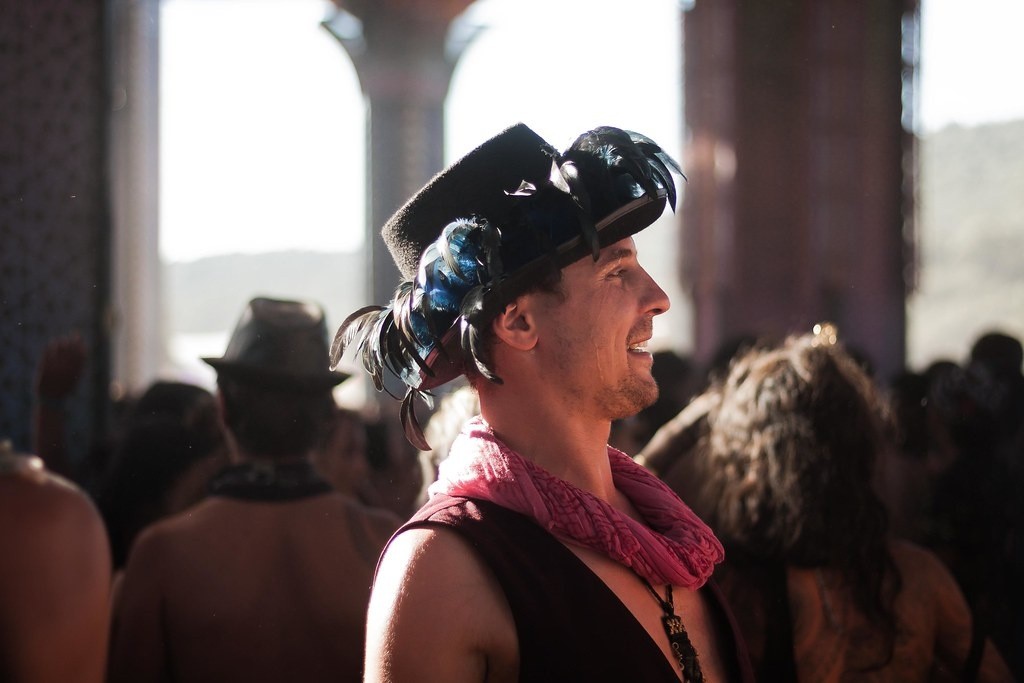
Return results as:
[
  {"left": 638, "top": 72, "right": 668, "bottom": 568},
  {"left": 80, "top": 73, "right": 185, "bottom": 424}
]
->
[
  {"left": 202, "top": 297, "right": 352, "bottom": 386},
  {"left": 332, "top": 124, "right": 687, "bottom": 452},
  {"left": 957, "top": 331, "right": 1024, "bottom": 382}
]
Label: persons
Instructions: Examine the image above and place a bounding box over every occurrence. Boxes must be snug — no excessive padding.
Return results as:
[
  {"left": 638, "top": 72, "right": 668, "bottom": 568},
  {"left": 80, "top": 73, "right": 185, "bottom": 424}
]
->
[
  {"left": 329, "top": 122, "right": 751, "bottom": 683},
  {"left": 0, "top": 296, "right": 482, "bottom": 683},
  {"left": 610, "top": 330, "right": 1024, "bottom": 683}
]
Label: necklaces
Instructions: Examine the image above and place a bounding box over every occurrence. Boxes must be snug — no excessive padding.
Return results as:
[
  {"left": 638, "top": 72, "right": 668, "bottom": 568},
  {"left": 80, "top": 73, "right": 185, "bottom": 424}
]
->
[{"left": 642, "top": 575, "right": 704, "bottom": 683}]
